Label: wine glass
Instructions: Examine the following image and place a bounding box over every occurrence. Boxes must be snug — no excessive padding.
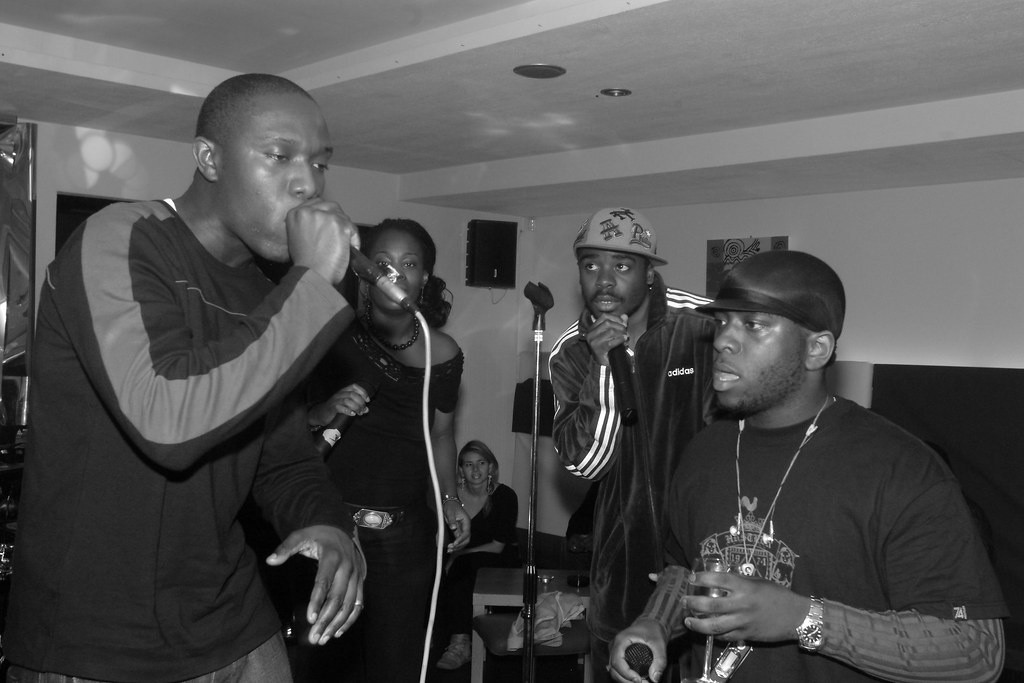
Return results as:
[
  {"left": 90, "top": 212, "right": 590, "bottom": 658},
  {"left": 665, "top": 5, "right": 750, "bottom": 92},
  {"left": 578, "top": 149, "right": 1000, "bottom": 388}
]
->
[{"left": 679, "top": 558, "right": 741, "bottom": 683}]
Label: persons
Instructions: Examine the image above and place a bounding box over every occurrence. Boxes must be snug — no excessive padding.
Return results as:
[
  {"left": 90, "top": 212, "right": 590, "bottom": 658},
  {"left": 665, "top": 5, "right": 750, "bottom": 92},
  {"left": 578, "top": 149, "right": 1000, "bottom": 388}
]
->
[
  {"left": 433, "top": 440, "right": 519, "bottom": 669},
  {"left": 542, "top": 205, "right": 718, "bottom": 683},
  {"left": 604, "top": 250, "right": 1008, "bottom": 683},
  {"left": 0, "top": 72, "right": 368, "bottom": 682},
  {"left": 293, "top": 217, "right": 471, "bottom": 683}
]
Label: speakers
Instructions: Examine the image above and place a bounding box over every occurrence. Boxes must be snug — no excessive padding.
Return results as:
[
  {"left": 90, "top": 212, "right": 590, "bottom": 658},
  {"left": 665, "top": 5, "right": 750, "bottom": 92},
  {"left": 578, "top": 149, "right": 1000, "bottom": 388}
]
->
[{"left": 465, "top": 219, "right": 518, "bottom": 289}]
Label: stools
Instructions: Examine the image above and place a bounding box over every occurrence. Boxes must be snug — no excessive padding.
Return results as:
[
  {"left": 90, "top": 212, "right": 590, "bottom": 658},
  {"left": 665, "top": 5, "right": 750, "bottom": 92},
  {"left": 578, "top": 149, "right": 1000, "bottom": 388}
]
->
[{"left": 471, "top": 614, "right": 590, "bottom": 683}]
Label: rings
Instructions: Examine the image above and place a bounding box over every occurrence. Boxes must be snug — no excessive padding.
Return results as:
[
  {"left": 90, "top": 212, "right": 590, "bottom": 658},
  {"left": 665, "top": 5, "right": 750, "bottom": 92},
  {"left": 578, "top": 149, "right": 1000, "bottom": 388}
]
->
[
  {"left": 355, "top": 600, "right": 365, "bottom": 608},
  {"left": 606, "top": 666, "right": 611, "bottom": 671}
]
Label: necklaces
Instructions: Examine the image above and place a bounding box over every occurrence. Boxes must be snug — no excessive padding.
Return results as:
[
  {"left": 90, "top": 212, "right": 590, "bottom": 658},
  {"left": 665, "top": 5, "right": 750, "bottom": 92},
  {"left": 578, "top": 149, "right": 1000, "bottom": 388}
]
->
[
  {"left": 733, "top": 395, "right": 830, "bottom": 576},
  {"left": 367, "top": 304, "right": 420, "bottom": 351}
]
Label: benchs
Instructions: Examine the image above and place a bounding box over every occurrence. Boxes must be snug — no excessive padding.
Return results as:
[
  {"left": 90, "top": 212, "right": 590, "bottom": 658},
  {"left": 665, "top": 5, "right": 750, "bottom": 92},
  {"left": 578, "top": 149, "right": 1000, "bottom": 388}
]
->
[{"left": 473, "top": 527, "right": 567, "bottom": 570}]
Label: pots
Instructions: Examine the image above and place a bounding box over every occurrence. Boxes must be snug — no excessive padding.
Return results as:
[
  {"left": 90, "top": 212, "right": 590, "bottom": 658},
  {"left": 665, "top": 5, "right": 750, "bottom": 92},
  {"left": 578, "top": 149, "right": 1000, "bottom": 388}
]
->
[{"left": 537, "top": 575, "right": 554, "bottom": 584}]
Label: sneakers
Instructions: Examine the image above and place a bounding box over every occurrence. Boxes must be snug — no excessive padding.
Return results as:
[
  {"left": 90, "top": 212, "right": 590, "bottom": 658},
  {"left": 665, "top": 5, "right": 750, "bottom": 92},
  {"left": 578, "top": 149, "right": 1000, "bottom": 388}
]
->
[{"left": 437, "top": 634, "right": 471, "bottom": 669}]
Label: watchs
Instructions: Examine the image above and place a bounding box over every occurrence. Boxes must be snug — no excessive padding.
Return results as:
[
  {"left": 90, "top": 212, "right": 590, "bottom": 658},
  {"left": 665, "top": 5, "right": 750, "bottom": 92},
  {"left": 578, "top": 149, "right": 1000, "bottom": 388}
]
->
[{"left": 797, "top": 595, "right": 824, "bottom": 653}]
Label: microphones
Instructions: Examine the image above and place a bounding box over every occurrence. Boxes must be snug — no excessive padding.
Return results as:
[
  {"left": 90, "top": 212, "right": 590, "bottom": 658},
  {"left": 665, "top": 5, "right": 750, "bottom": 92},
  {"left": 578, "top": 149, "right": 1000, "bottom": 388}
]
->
[
  {"left": 624, "top": 643, "right": 653, "bottom": 681},
  {"left": 346, "top": 245, "right": 420, "bottom": 315},
  {"left": 607, "top": 343, "right": 640, "bottom": 428},
  {"left": 315, "top": 381, "right": 377, "bottom": 458}
]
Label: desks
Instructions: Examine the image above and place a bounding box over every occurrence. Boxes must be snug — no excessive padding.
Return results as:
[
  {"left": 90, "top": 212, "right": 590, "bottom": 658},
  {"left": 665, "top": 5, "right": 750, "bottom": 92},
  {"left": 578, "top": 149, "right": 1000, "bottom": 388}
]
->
[{"left": 470, "top": 567, "right": 591, "bottom": 683}]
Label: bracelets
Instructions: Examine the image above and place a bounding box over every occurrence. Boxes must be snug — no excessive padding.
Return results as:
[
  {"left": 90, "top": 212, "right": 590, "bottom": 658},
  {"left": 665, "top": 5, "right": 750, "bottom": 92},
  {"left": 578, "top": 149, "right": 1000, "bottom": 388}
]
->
[
  {"left": 306, "top": 425, "right": 323, "bottom": 431},
  {"left": 442, "top": 494, "right": 464, "bottom": 504}
]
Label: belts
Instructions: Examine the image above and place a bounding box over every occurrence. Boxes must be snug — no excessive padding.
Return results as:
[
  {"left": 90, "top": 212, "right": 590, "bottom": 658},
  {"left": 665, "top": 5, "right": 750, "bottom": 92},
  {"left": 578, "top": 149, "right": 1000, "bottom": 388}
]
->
[{"left": 347, "top": 506, "right": 420, "bottom": 530}]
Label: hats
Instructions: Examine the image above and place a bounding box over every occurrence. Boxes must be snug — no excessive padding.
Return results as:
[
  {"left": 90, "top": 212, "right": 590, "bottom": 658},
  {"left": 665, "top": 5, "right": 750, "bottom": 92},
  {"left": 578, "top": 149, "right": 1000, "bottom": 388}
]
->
[
  {"left": 572, "top": 206, "right": 668, "bottom": 267},
  {"left": 694, "top": 250, "right": 846, "bottom": 344}
]
